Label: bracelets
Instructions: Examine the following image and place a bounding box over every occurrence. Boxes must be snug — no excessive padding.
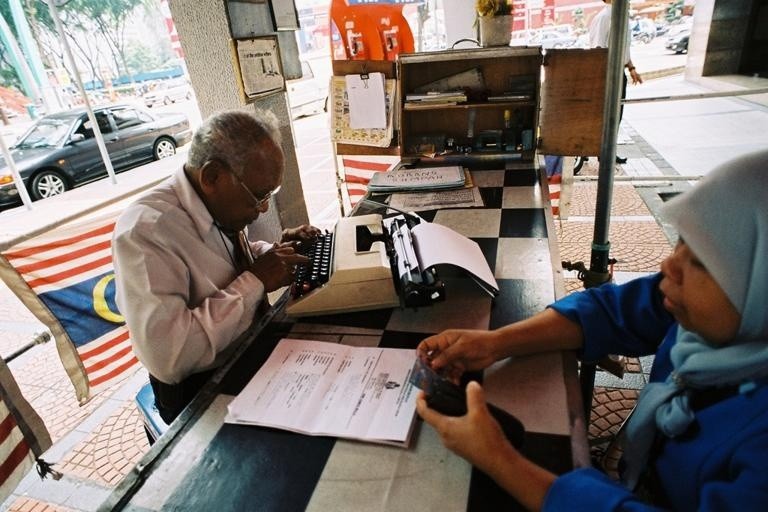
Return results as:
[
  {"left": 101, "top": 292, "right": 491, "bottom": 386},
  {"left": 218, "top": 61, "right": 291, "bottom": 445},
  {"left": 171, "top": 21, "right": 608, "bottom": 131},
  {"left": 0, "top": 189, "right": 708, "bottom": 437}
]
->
[{"left": 629, "top": 66, "right": 636, "bottom": 73}]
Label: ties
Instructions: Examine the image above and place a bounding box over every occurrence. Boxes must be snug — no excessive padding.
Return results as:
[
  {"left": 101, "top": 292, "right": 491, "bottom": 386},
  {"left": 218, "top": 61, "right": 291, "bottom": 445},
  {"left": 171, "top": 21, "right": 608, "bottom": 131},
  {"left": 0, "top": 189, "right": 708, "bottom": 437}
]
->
[{"left": 218, "top": 229, "right": 272, "bottom": 317}]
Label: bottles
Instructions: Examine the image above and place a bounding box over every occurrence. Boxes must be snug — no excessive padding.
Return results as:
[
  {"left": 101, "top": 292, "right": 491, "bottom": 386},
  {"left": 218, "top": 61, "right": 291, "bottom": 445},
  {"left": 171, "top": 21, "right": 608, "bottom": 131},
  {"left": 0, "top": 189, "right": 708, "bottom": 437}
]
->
[
  {"left": 502, "top": 109, "right": 512, "bottom": 130},
  {"left": 446, "top": 138, "right": 453, "bottom": 150}
]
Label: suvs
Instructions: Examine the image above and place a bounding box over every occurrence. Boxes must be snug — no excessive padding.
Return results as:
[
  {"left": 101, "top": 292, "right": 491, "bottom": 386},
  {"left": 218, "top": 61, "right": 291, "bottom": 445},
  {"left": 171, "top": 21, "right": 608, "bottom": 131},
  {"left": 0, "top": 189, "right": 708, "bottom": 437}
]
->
[{"left": 142, "top": 77, "right": 194, "bottom": 108}]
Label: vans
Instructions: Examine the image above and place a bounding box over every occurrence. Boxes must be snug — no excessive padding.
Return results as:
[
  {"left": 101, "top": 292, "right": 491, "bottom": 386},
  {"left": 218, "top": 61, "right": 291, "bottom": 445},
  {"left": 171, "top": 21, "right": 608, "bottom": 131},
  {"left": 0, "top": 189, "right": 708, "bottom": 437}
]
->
[{"left": 285, "top": 48, "right": 332, "bottom": 121}]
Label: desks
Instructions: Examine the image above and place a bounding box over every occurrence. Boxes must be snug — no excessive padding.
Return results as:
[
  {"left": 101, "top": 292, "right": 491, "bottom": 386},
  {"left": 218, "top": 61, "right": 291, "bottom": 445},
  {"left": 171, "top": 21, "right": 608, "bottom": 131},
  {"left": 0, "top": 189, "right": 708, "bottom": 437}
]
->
[{"left": 98, "top": 102, "right": 581, "bottom": 510}]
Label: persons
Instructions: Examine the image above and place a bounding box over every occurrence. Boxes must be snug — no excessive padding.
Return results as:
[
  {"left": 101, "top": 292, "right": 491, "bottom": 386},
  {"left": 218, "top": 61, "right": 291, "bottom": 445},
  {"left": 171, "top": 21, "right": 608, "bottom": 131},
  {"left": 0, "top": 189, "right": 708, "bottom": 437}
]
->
[
  {"left": 589, "top": 1, "right": 645, "bottom": 164},
  {"left": 106, "top": 108, "right": 326, "bottom": 424},
  {"left": 411, "top": 145, "right": 768, "bottom": 510}
]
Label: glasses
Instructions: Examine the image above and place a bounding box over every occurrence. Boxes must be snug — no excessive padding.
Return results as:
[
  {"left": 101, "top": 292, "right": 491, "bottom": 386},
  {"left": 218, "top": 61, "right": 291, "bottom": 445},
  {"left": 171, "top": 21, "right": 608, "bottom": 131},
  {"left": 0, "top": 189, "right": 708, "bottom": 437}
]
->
[{"left": 208, "top": 157, "right": 283, "bottom": 210}]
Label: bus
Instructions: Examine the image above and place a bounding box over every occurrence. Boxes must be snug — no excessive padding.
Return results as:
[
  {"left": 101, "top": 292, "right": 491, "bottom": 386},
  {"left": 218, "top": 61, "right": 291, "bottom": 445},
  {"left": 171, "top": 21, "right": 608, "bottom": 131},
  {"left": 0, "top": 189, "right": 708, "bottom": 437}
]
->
[{"left": 510, "top": 24, "right": 576, "bottom": 46}]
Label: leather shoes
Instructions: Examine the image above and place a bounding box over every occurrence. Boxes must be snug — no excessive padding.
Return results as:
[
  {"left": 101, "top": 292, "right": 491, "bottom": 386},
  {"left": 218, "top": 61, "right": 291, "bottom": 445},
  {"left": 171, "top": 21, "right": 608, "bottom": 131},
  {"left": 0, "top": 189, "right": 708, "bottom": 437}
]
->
[
  {"left": 573, "top": 155, "right": 584, "bottom": 175},
  {"left": 615, "top": 156, "right": 629, "bottom": 164}
]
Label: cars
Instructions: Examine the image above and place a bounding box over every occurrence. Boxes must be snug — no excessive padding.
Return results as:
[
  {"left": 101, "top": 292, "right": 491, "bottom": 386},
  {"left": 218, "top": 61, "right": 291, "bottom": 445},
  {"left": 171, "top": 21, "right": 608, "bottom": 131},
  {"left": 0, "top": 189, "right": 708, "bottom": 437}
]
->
[
  {"left": 630, "top": 16, "right": 692, "bottom": 55},
  {"left": 524, "top": 30, "right": 576, "bottom": 49},
  {"left": 0, "top": 102, "right": 194, "bottom": 213}
]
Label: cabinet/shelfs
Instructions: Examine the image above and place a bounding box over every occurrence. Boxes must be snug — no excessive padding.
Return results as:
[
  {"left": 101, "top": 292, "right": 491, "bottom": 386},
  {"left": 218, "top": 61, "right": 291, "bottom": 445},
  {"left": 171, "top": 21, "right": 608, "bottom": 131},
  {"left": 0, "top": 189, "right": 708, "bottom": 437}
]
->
[{"left": 392, "top": 40, "right": 548, "bottom": 161}]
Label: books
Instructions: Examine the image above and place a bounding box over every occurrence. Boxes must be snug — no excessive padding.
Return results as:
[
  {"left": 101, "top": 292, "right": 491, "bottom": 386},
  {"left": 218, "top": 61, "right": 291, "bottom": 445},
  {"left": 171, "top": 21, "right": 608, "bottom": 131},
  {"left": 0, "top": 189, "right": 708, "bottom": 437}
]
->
[{"left": 401, "top": 88, "right": 468, "bottom": 110}]
